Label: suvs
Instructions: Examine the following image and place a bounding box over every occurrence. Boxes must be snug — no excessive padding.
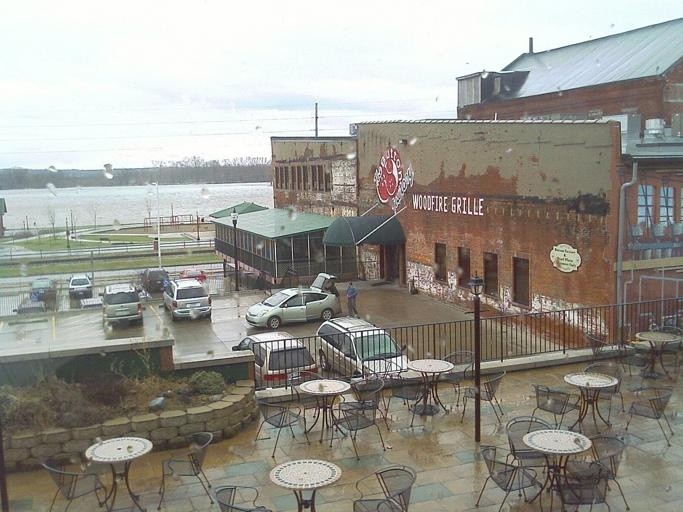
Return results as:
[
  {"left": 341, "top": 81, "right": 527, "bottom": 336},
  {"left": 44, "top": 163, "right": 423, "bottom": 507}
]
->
[
  {"left": 230, "top": 330, "right": 318, "bottom": 387},
  {"left": 139, "top": 268, "right": 169, "bottom": 293},
  {"left": 66, "top": 273, "right": 94, "bottom": 298},
  {"left": 160, "top": 277, "right": 212, "bottom": 321},
  {"left": 314, "top": 316, "right": 413, "bottom": 380},
  {"left": 97, "top": 283, "right": 145, "bottom": 324}
]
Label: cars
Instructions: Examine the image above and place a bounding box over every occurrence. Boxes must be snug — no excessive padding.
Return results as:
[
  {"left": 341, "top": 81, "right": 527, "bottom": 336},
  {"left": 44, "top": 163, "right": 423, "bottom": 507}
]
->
[
  {"left": 77, "top": 295, "right": 101, "bottom": 310},
  {"left": 28, "top": 275, "right": 55, "bottom": 301},
  {"left": 243, "top": 271, "right": 342, "bottom": 331},
  {"left": 12, "top": 302, "right": 53, "bottom": 315},
  {"left": 177, "top": 268, "right": 206, "bottom": 281}
]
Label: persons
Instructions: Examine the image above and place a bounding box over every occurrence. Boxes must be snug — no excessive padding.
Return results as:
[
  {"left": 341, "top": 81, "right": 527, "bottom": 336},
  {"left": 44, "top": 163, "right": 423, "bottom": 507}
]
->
[
  {"left": 198, "top": 271, "right": 206, "bottom": 282},
  {"left": 324, "top": 281, "right": 342, "bottom": 314},
  {"left": 345, "top": 283, "right": 358, "bottom": 318}
]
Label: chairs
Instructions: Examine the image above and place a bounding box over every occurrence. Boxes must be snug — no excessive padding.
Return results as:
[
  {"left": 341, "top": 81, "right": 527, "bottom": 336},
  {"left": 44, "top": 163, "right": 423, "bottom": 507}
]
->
[
  {"left": 157, "top": 432, "right": 214, "bottom": 510},
  {"left": 210, "top": 485, "right": 273, "bottom": 511},
  {"left": 40, "top": 450, "right": 109, "bottom": 512}
]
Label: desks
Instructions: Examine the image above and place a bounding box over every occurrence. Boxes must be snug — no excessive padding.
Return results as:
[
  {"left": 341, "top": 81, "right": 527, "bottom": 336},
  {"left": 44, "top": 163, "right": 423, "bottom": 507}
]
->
[{"left": 87, "top": 436, "right": 154, "bottom": 511}]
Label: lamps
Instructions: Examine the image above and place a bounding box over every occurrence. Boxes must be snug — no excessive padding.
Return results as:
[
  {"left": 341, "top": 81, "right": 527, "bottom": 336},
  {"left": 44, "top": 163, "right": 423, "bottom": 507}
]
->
[{"left": 399, "top": 139, "right": 407, "bottom": 145}]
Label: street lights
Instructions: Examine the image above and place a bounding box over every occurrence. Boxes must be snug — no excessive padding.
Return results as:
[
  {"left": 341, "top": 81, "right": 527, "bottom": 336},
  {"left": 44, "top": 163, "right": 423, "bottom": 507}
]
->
[
  {"left": 64, "top": 217, "right": 70, "bottom": 249},
  {"left": 465, "top": 271, "right": 491, "bottom": 442},
  {"left": 230, "top": 207, "right": 240, "bottom": 292},
  {"left": 147, "top": 181, "right": 161, "bottom": 270}
]
